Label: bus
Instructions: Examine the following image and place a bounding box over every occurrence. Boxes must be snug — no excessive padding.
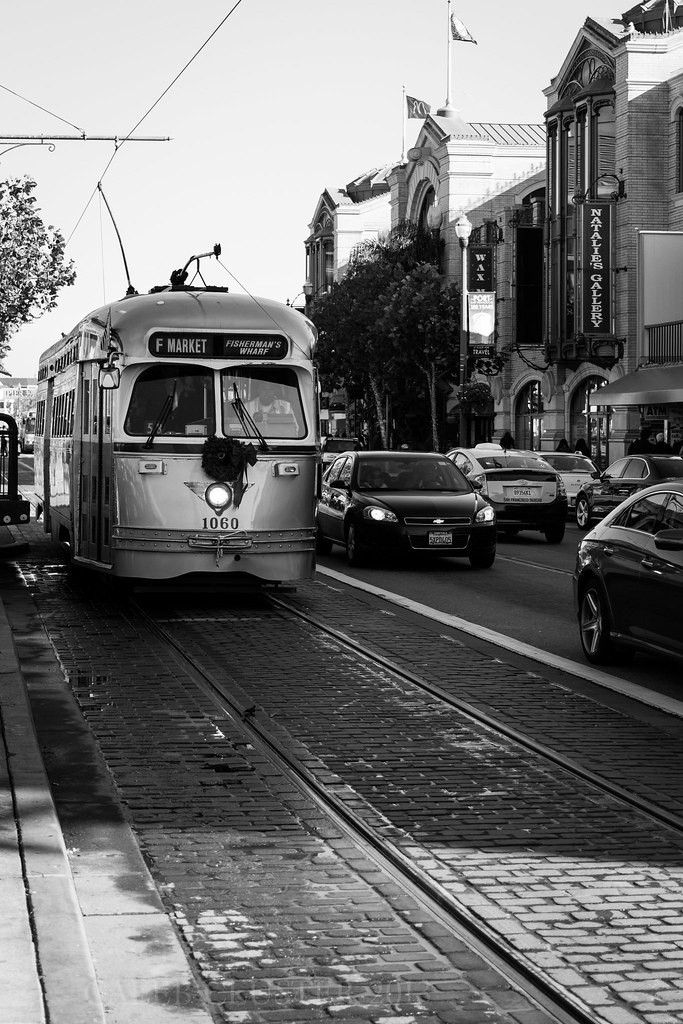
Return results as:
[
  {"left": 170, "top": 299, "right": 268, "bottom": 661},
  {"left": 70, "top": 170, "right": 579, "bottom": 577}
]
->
[
  {"left": 32, "top": 181, "right": 325, "bottom": 594},
  {"left": 19, "top": 411, "right": 36, "bottom": 454}
]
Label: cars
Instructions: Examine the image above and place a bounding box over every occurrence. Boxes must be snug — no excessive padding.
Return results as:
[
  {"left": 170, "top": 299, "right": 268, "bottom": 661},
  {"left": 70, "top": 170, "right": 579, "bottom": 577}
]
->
[
  {"left": 0, "top": 427, "right": 21, "bottom": 453},
  {"left": 574, "top": 454, "right": 683, "bottom": 528},
  {"left": 320, "top": 444, "right": 497, "bottom": 574},
  {"left": 531, "top": 450, "right": 603, "bottom": 520},
  {"left": 573, "top": 478, "right": 683, "bottom": 676},
  {"left": 445, "top": 442, "right": 568, "bottom": 543}
]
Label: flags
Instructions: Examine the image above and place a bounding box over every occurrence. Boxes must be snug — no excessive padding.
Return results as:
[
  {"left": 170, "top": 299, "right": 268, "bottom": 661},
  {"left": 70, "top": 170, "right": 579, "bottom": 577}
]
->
[
  {"left": 451, "top": 13, "right": 479, "bottom": 45},
  {"left": 405, "top": 95, "right": 432, "bottom": 120}
]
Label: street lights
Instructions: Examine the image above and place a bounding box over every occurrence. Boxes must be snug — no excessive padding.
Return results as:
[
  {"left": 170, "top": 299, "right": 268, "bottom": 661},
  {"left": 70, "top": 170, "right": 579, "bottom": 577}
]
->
[{"left": 455, "top": 212, "right": 472, "bottom": 444}]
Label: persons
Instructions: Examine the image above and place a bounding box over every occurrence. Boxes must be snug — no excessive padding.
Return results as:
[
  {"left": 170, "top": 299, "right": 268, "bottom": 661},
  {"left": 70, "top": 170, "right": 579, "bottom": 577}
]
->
[
  {"left": 653, "top": 433, "right": 675, "bottom": 455},
  {"left": 410, "top": 467, "right": 443, "bottom": 489},
  {"left": 323, "top": 430, "right": 464, "bottom": 458},
  {"left": 362, "top": 467, "right": 390, "bottom": 490},
  {"left": 576, "top": 439, "right": 593, "bottom": 460},
  {"left": 236, "top": 378, "right": 299, "bottom": 435},
  {"left": 500, "top": 432, "right": 516, "bottom": 451},
  {"left": 629, "top": 429, "right": 657, "bottom": 456},
  {"left": 555, "top": 440, "right": 572, "bottom": 454}
]
